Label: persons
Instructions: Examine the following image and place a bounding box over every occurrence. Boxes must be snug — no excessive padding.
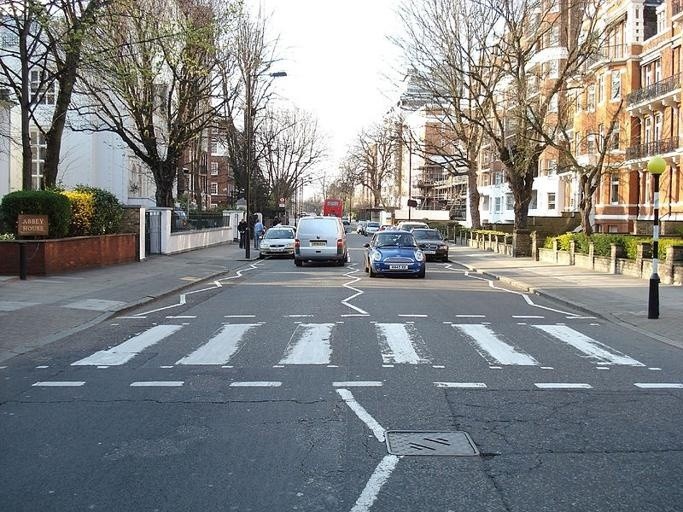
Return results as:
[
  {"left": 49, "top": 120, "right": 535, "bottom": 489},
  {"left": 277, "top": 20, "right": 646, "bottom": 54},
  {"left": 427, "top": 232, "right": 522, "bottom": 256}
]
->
[
  {"left": 254, "top": 219, "right": 263, "bottom": 250},
  {"left": 236, "top": 220, "right": 246, "bottom": 249}
]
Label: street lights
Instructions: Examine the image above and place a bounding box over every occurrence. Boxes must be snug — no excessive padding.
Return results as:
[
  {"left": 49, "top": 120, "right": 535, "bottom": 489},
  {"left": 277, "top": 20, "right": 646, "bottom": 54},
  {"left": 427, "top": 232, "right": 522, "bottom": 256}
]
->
[
  {"left": 246, "top": 72, "right": 287, "bottom": 218},
  {"left": 647, "top": 157, "right": 665, "bottom": 317}
]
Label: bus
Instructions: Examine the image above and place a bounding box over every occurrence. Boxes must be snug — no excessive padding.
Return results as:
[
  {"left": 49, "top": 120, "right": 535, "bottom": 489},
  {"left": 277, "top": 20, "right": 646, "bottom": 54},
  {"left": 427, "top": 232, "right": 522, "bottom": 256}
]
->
[{"left": 324, "top": 198, "right": 342, "bottom": 217}]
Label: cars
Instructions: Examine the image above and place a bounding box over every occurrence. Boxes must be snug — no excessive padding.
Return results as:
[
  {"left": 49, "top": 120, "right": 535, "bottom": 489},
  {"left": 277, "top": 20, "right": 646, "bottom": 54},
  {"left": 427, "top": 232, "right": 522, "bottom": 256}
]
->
[
  {"left": 259, "top": 225, "right": 296, "bottom": 259},
  {"left": 363, "top": 231, "right": 425, "bottom": 278},
  {"left": 377, "top": 224, "right": 396, "bottom": 231},
  {"left": 342, "top": 217, "right": 350, "bottom": 225}
]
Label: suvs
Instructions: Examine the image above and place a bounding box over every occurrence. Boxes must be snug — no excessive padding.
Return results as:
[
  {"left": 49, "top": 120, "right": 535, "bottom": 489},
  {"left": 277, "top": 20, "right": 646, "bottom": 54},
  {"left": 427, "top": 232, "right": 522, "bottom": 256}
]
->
[
  {"left": 356, "top": 221, "right": 380, "bottom": 236},
  {"left": 410, "top": 227, "right": 449, "bottom": 261},
  {"left": 397, "top": 221, "right": 430, "bottom": 232}
]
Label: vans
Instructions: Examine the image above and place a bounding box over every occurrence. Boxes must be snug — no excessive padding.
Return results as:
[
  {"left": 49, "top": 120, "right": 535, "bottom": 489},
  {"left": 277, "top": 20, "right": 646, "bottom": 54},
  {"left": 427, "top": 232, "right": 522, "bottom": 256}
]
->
[{"left": 294, "top": 216, "right": 348, "bottom": 266}]
